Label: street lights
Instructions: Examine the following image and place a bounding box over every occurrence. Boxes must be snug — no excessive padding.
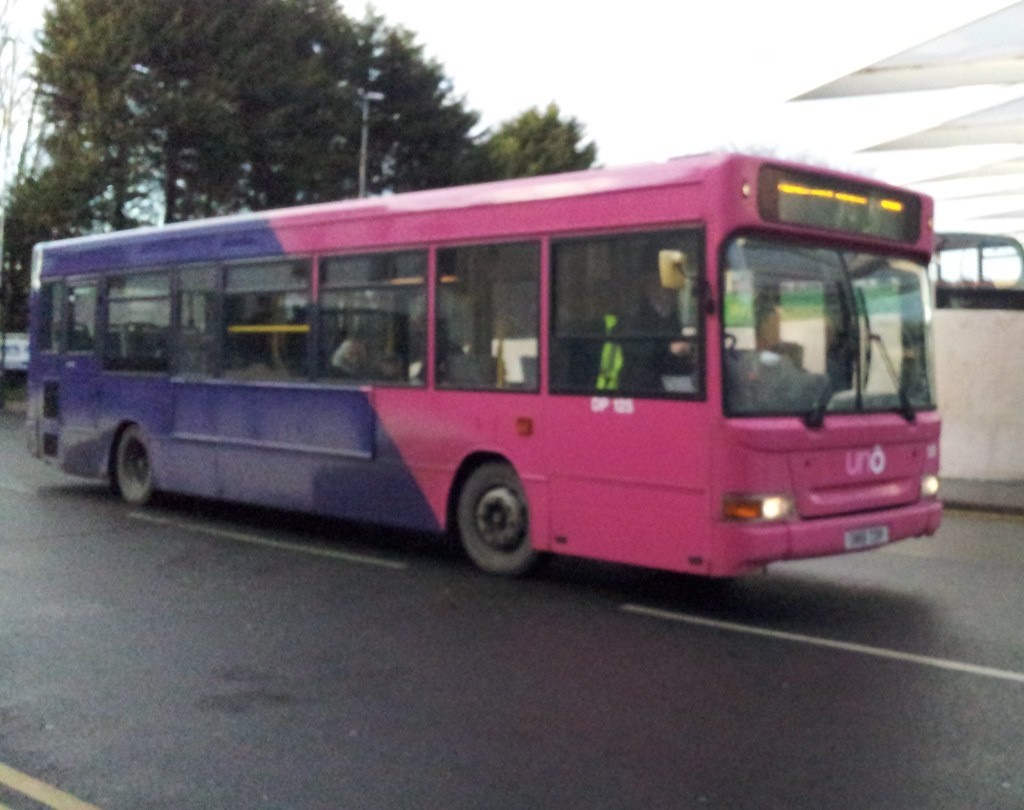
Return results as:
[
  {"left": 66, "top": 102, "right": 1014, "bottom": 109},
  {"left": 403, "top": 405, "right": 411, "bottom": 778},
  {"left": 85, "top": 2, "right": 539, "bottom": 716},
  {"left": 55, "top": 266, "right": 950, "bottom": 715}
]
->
[{"left": 356, "top": 91, "right": 384, "bottom": 196}]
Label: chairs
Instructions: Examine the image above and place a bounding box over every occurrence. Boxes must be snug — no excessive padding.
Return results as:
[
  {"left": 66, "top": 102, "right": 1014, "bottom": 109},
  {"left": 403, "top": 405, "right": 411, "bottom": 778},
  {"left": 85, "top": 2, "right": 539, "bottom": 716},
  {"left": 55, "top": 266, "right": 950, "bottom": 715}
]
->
[{"left": 48, "top": 320, "right": 496, "bottom": 391}]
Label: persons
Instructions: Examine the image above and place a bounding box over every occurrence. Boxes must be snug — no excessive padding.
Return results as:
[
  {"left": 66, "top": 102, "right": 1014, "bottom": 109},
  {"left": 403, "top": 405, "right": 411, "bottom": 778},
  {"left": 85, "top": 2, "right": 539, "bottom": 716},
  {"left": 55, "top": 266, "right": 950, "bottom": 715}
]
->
[
  {"left": 331, "top": 337, "right": 370, "bottom": 379},
  {"left": 622, "top": 278, "right": 700, "bottom": 377},
  {"left": 751, "top": 304, "right": 803, "bottom": 370}
]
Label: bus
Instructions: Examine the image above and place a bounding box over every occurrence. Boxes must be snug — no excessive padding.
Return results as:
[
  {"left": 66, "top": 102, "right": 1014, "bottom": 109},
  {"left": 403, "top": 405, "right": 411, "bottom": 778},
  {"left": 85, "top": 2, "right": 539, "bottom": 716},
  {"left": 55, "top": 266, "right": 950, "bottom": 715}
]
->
[{"left": 22, "top": 148, "right": 943, "bottom": 576}]
